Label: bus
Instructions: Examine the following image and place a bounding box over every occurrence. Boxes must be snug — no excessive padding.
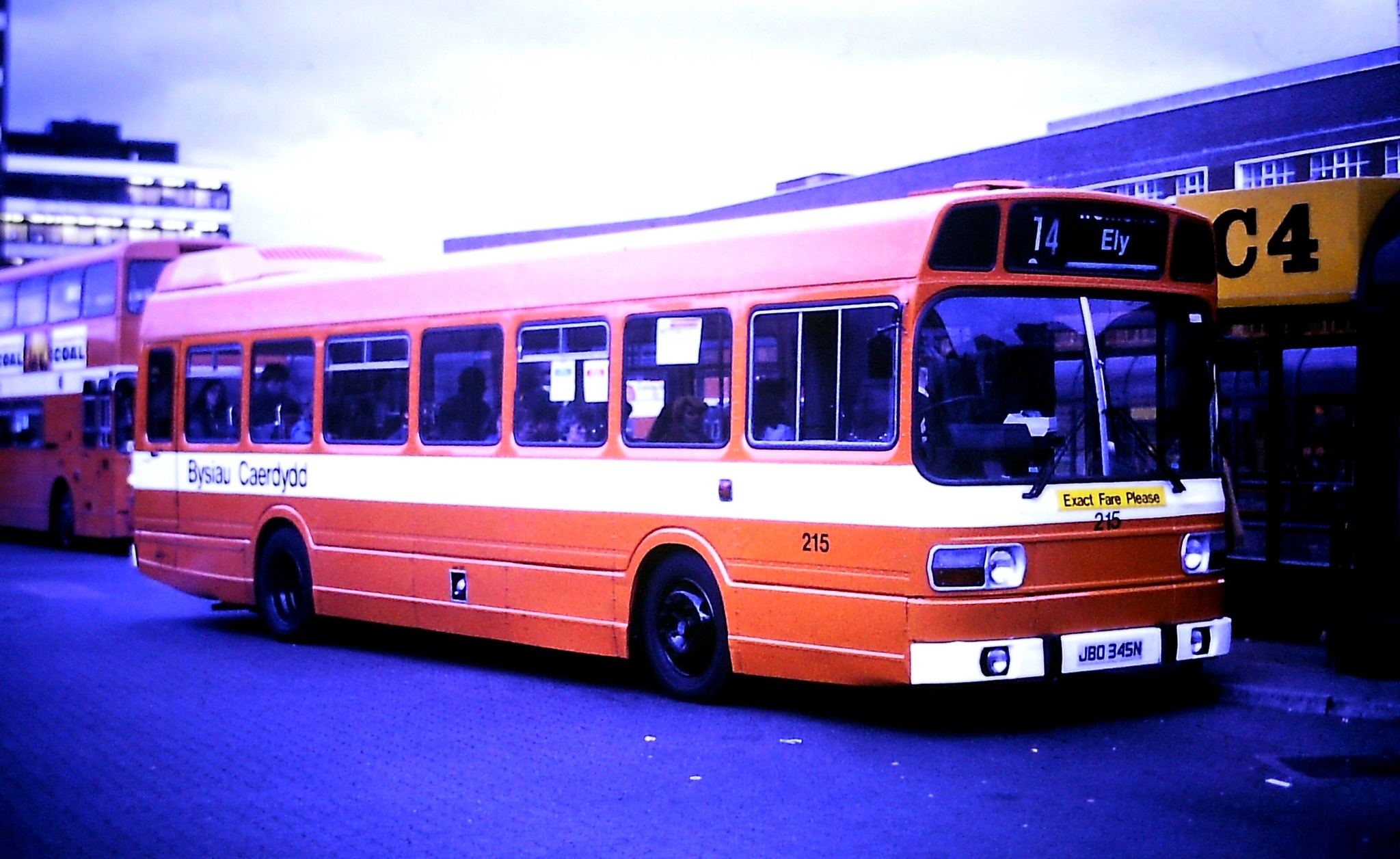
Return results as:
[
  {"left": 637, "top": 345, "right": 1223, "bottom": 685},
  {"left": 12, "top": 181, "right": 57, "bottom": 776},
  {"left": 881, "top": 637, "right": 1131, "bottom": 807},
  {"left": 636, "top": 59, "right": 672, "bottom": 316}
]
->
[
  {"left": 0, "top": 234, "right": 254, "bottom": 551},
  {"left": 128, "top": 178, "right": 1241, "bottom": 704}
]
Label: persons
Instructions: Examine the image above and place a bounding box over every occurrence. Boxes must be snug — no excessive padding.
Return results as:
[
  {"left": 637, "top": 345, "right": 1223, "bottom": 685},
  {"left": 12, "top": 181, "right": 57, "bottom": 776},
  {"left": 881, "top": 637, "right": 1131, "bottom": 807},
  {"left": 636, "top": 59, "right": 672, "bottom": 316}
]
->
[
  {"left": 190, "top": 377, "right": 238, "bottom": 437},
  {"left": 252, "top": 362, "right": 302, "bottom": 425},
  {"left": 496, "top": 405, "right": 587, "bottom": 444},
  {"left": 659, "top": 396, "right": 713, "bottom": 444},
  {"left": 437, "top": 367, "right": 491, "bottom": 441}
]
